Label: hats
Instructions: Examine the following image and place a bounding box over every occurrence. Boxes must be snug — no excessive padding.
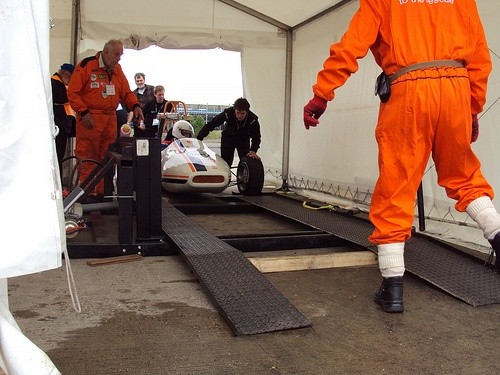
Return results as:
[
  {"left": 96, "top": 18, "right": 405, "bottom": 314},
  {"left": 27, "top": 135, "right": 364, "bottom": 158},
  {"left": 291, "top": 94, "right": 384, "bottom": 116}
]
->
[{"left": 61, "top": 63, "right": 74, "bottom": 75}]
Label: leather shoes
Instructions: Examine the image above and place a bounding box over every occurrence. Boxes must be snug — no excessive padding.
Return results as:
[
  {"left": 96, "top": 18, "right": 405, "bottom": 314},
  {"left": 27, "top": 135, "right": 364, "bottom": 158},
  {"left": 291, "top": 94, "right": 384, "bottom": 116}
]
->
[
  {"left": 373, "top": 276, "right": 403, "bottom": 313},
  {"left": 488, "top": 232, "right": 500, "bottom": 272}
]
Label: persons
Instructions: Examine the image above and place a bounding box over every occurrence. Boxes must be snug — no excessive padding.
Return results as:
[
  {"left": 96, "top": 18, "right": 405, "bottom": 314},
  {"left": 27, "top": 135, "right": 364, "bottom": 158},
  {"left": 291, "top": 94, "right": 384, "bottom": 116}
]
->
[
  {"left": 196, "top": 97, "right": 262, "bottom": 169},
  {"left": 51, "top": 38, "right": 174, "bottom": 203},
  {"left": 302, "top": 0, "right": 500, "bottom": 314}
]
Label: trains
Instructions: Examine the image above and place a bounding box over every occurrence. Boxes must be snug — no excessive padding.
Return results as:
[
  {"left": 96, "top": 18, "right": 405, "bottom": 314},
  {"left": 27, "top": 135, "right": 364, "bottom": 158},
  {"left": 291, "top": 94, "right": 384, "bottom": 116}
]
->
[{"left": 177, "top": 108, "right": 206, "bottom": 112}]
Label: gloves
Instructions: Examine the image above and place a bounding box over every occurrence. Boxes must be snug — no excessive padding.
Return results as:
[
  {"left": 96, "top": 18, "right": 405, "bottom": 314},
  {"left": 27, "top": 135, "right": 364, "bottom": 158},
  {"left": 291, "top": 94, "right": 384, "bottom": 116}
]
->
[
  {"left": 303, "top": 97, "right": 327, "bottom": 130},
  {"left": 472, "top": 115, "right": 479, "bottom": 142}
]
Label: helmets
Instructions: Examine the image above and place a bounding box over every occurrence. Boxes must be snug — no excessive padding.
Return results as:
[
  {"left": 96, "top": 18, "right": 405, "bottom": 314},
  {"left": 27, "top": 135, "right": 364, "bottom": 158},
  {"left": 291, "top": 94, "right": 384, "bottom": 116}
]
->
[{"left": 172, "top": 120, "right": 194, "bottom": 139}]
